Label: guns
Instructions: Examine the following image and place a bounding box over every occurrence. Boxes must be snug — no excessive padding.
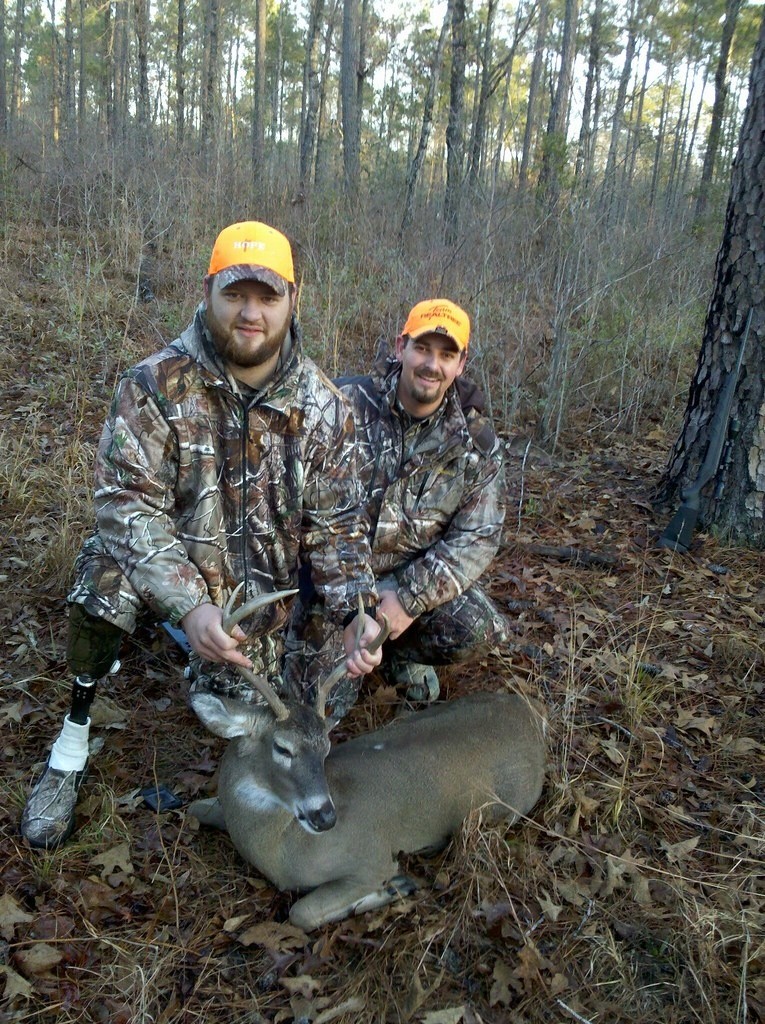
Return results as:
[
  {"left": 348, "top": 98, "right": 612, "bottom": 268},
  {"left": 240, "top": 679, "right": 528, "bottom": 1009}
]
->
[{"left": 657, "top": 306, "right": 754, "bottom": 552}]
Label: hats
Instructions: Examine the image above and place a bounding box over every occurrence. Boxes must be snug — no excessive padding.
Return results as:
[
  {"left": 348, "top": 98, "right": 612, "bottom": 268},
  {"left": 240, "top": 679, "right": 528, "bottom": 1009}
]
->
[
  {"left": 208, "top": 221, "right": 293, "bottom": 297},
  {"left": 402, "top": 298, "right": 470, "bottom": 354}
]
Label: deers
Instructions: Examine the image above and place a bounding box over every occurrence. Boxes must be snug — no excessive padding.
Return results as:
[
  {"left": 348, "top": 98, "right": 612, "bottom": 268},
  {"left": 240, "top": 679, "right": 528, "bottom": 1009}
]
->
[{"left": 185, "top": 581, "right": 550, "bottom": 932}]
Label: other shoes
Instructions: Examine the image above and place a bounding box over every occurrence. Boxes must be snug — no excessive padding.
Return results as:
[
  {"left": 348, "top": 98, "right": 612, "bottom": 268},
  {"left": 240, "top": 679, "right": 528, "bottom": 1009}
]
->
[{"left": 391, "top": 661, "right": 440, "bottom": 704}]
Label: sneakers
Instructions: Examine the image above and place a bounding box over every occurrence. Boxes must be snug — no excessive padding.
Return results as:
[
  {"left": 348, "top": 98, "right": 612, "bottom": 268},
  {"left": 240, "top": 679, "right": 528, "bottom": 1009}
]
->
[{"left": 21, "top": 753, "right": 89, "bottom": 848}]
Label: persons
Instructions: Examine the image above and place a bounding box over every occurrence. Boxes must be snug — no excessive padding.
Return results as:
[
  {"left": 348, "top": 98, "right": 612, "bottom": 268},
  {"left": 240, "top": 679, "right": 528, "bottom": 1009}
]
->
[
  {"left": 273, "top": 298, "right": 508, "bottom": 702},
  {"left": 20, "top": 222, "right": 383, "bottom": 846}
]
